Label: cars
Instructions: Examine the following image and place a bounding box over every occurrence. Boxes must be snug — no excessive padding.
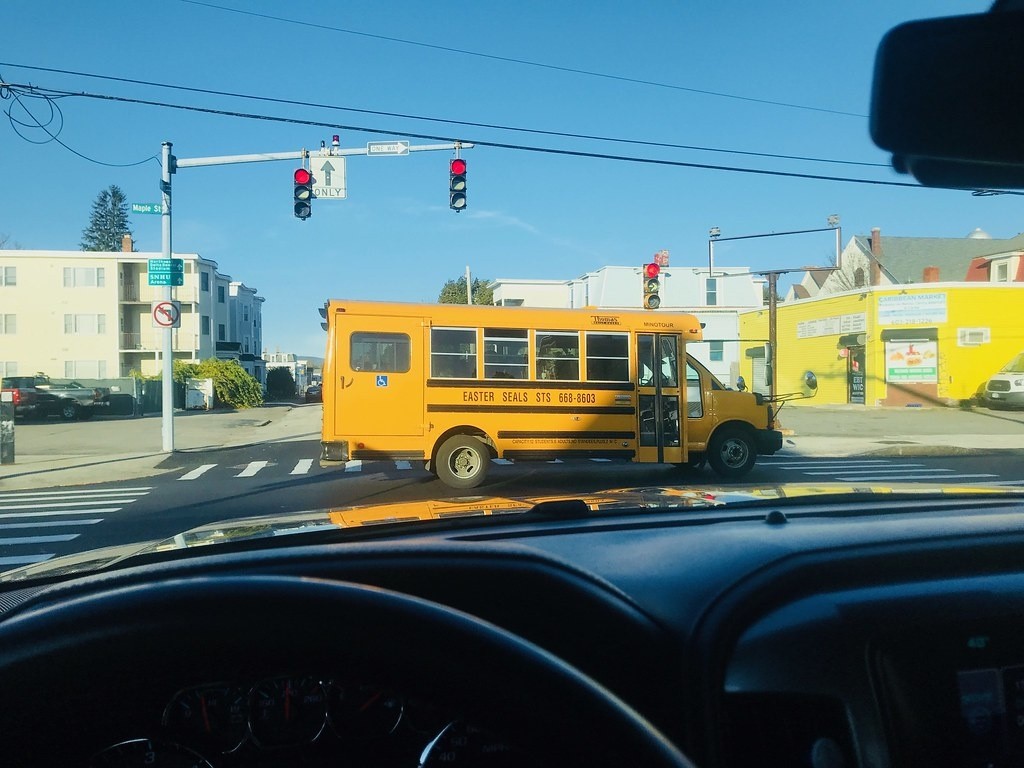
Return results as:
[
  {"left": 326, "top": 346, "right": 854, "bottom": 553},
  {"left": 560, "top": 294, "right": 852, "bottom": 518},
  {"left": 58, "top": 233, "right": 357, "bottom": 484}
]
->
[
  {"left": 0, "top": 377, "right": 55, "bottom": 420},
  {"left": 983, "top": 352, "right": 1024, "bottom": 410}
]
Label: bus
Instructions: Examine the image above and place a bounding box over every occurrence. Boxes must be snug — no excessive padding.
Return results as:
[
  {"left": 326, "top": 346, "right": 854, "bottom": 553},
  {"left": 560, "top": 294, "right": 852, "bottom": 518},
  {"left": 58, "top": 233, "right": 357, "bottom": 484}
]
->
[{"left": 316, "top": 300, "right": 818, "bottom": 489}]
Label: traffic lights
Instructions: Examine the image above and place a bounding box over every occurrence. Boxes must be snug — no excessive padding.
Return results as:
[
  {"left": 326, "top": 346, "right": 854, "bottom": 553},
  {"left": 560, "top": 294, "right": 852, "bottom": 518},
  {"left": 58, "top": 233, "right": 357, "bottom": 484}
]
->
[
  {"left": 450, "top": 159, "right": 467, "bottom": 212},
  {"left": 643, "top": 263, "right": 662, "bottom": 311},
  {"left": 293, "top": 167, "right": 312, "bottom": 220}
]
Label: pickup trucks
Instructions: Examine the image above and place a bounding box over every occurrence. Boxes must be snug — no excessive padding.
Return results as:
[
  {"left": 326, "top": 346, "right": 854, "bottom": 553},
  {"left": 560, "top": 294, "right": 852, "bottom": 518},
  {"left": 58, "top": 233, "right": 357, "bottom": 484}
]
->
[{"left": 34, "top": 376, "right": 111, "bottom": 420}]
